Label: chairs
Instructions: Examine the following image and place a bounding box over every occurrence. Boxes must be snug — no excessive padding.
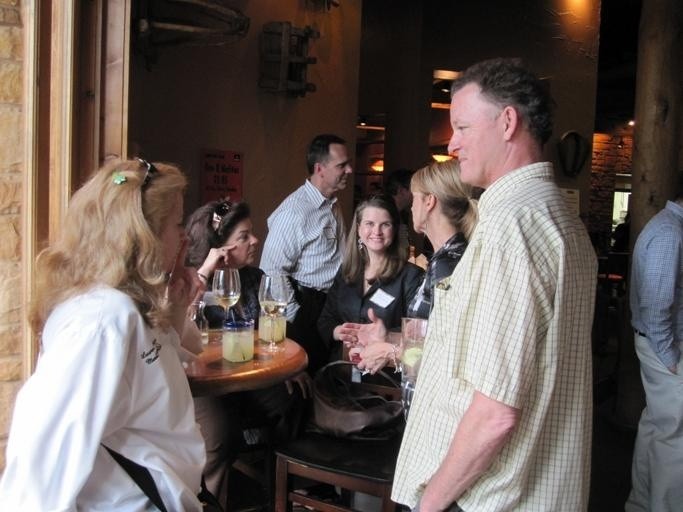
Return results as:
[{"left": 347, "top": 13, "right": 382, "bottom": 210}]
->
[{"left": 270, "top": 425, "right": 396, "bottom": 512}]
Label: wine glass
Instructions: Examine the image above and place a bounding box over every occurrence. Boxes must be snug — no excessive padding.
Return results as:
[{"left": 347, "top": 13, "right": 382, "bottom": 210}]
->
[
  {"left": 212, "top": 267, "right": 241, "bottom": 345},
  {"left": 258, "top": 271, "right": 293, "bottom": 354}
]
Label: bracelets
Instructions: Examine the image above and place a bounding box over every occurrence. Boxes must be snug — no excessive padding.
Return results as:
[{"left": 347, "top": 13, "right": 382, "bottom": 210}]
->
[{"left": 195, "top": 272, "right": 209, "bottom": 282}]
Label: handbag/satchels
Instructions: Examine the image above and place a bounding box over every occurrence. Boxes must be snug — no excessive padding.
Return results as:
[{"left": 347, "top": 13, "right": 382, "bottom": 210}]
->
[{"left": 304, "top": 360, "right": 405, "bottom": 442}]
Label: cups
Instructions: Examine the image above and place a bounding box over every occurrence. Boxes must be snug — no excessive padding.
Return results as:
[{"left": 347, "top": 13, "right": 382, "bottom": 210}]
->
[
  {"left": 221, "top": 318, "right": 256, "bottom": 362},
  {"left": 257, "top": 301, "right": 288, "bottom": 344}
]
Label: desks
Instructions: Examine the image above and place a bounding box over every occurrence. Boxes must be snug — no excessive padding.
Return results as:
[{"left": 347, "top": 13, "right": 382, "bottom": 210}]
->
[{"left": 185, "top": 329, "right": 309, "bottom": 512}]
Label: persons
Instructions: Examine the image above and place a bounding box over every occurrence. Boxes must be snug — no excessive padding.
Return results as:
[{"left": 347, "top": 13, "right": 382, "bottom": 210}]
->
[
  {"left": 314, "top": 162, "right": 482, "bottom": 512},
  {"left": 383, "top": 52, "right": 603, "bottom": 512},
  {"left": 621, "top": 194, "right": 683, "bottom": 512},
  {"left": 607, "top": 211, "right": 631, "bottom": 300},
  {"left": 0, "top": 152, "right": 210, "bottom": 511},
  {"left": 171, "top": 196, "right": 312, "bottom": 510},
  {"left": 256, "top": 131, "right": 355, "bottom": 364}
]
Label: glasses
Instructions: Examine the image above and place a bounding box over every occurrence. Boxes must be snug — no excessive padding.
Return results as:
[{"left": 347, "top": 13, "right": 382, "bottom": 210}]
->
[
  {"left": 213, "top": 201, "right": 233, "bottom": 232},
  {"left": 137, "top": 158, "right": 158, "bottom": 191}
]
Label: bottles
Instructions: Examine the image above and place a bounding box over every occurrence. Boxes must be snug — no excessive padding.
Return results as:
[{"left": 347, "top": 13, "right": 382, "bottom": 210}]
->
[{"left": 189, "top": 300, "right": 209, "bottom": 345}]
